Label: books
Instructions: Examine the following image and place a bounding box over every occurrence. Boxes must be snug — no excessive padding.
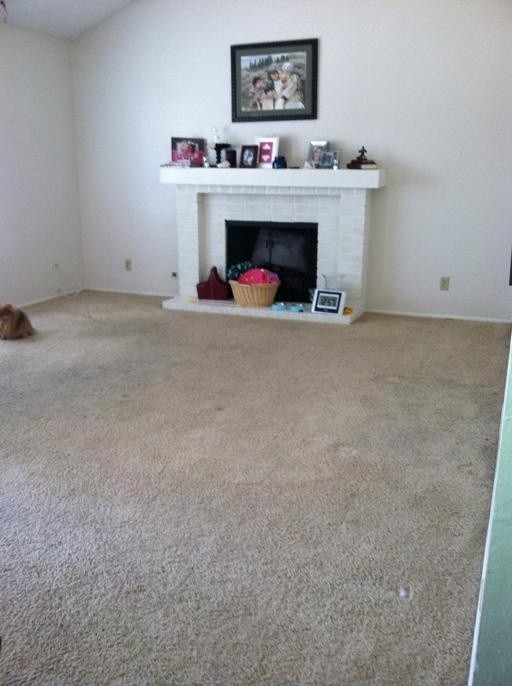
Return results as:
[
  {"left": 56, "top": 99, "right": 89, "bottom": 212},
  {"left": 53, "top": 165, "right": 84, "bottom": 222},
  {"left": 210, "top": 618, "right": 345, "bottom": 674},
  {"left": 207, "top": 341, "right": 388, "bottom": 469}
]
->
[{"left": 347, "top": 160, "right": 378, "bottom": 169}]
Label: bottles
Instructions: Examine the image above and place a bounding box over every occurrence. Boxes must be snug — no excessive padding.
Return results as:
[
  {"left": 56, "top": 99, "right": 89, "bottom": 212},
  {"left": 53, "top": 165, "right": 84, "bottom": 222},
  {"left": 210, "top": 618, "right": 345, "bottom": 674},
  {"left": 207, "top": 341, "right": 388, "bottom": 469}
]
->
[
  {"left": 273, "top": 156, "right": 287, "bottom": 169},
  {"left": 333, "top": 158, "right": 339, "bottom": 169}
]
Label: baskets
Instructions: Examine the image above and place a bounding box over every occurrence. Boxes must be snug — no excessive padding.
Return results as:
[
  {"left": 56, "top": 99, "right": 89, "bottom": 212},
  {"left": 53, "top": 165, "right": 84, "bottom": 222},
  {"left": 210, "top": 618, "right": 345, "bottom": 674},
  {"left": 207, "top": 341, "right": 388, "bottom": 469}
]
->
[{"left": 228, "top": 279, "right": 280, "bottom": 307}]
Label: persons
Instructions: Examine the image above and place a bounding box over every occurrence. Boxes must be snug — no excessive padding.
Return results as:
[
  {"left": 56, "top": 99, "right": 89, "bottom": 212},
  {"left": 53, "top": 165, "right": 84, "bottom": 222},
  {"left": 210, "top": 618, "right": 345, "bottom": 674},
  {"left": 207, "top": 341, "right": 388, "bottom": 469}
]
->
[
  {"left": 274, "top": 60, "right": 306, "bottom": 110},
  {"left": 241, "top": 146, "right": 256, "bottom": 167},
  {"left": 248, "top": 75, "right": 270, "bottom": 109},
  {"left": 261, "top": 68, "right": 306, "bottom": 110}
]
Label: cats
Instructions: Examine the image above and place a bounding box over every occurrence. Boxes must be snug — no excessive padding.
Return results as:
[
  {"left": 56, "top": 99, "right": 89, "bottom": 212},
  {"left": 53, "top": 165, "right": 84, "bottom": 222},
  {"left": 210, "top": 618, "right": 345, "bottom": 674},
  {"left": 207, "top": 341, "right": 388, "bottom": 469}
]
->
[{"left": 0, "top": 302, "right": 36, "bottom": 340}]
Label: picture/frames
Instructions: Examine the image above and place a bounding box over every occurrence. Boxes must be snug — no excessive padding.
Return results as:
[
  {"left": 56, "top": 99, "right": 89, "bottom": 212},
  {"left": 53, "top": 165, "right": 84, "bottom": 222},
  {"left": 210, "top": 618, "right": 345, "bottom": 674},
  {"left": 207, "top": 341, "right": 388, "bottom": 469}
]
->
[
  {"left": 230, "top": 37, "right": 319, "bottom": 123},
  {"left": 240, "top": 135, "right": 280, "bottom": 167},
  {"left": 171, "top": 135, "right": 208, "bottom": 165},
  {"left": 308, "top": 139, "right": 342, "bottom": 168}
]
